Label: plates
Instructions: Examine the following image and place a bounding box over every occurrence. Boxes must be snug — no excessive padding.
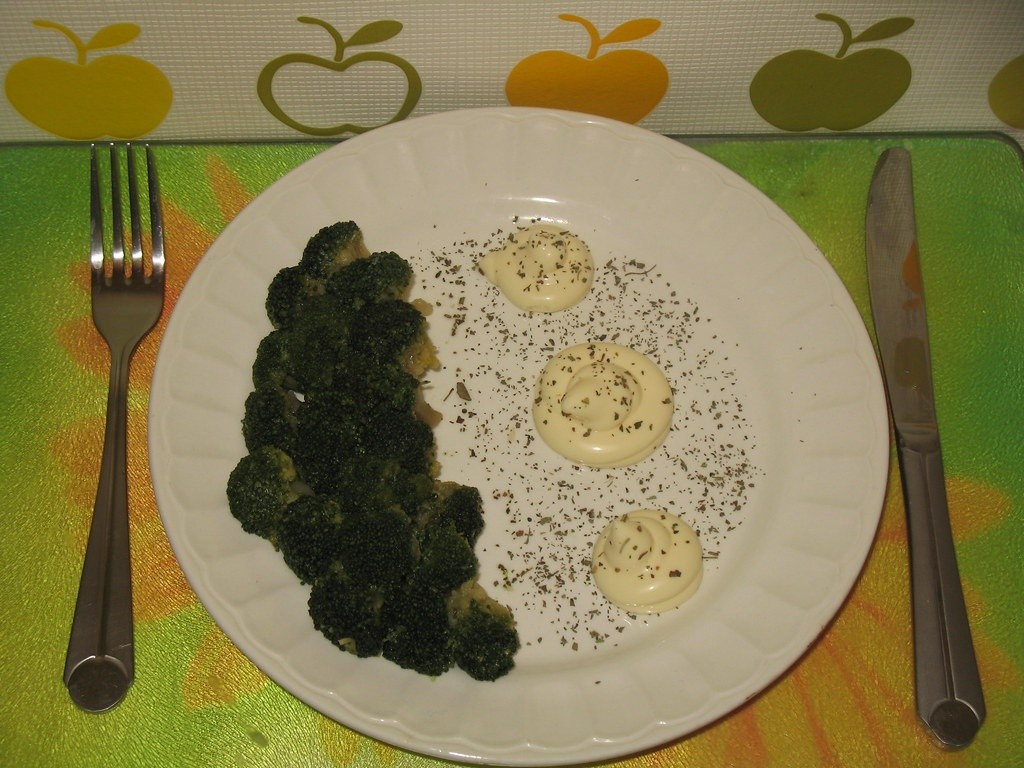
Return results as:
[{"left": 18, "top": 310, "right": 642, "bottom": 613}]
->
[{"left": 148, "top": 106, "right": 890, "bottom": 767}]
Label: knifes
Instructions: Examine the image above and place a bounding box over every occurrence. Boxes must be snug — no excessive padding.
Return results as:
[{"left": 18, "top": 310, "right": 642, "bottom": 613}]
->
[{"left": 865, "top": 147, "right": 987, "bottom": 752}]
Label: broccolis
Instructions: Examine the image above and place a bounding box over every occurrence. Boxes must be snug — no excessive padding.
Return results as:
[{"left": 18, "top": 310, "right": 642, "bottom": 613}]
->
[{"left": 227, "top": 221, "right": 518, "bottom": 681}]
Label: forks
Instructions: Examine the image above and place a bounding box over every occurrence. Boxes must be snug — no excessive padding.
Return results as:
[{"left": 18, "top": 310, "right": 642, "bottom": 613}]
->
[{"left": 63, "top": 143, "right": 167, "bottom": 715}]
